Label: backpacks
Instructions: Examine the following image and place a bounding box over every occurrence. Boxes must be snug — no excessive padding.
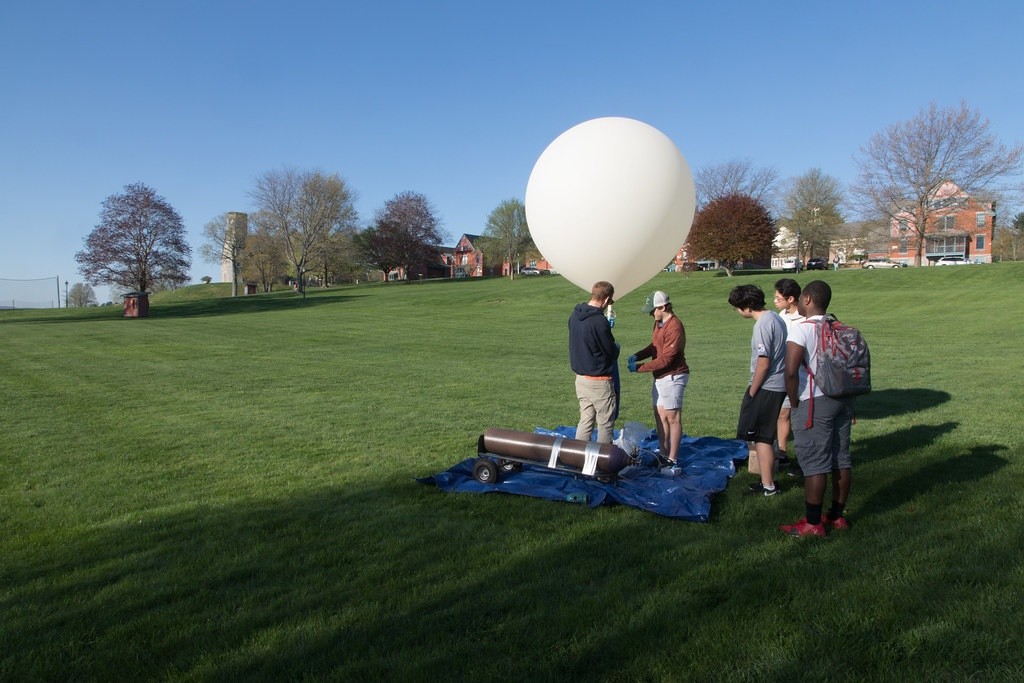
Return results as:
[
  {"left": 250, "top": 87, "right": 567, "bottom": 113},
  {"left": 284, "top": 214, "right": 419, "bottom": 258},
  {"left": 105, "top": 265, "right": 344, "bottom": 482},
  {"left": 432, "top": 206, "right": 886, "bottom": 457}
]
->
[{"left": 797, "top": 313, "right": 871, "bottom": 397}]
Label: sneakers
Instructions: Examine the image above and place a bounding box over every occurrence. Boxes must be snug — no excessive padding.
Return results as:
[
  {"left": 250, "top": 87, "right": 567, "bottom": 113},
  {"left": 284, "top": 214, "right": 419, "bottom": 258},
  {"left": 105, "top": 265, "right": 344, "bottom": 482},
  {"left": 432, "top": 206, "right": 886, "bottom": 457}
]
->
[
  {"left": 779, "top": 520, "right": 826, "bottom": 538},
  {"left": 749, "top": 482, "right": 775, "bottom": 491},
  {"left": 756, "top": 485, "right": 780, "bottom": 497},
  {"left": 802, "top": 515, "right": 848, "bottom": 531}
]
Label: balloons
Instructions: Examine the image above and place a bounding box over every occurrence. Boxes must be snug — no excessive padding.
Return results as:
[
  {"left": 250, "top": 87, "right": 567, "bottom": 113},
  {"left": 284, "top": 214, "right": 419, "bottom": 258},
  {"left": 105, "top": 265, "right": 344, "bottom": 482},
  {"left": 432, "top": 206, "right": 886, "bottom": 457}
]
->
[{"left": 524, "top": 116, "right": 697, "bottom": 304}]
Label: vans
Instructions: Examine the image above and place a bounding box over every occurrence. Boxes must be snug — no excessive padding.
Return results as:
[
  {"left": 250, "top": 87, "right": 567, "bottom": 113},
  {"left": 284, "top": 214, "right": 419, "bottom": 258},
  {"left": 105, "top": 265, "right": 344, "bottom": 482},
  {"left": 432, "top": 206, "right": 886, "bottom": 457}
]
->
[
  {"left": 455, "top": 267, "right": 469, "bottom": 278},
  {"left": 771, "top": 257, "right": 804, "bottom": 271}
]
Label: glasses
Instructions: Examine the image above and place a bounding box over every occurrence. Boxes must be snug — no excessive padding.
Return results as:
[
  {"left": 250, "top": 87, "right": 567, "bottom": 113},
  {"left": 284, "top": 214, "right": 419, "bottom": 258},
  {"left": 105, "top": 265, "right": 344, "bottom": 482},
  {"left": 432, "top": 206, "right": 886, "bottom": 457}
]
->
[
  {"left": 773, "top": 294, "right": 791, "bottom": 302},
  {"left": 605, "top": 296, "right": 614, "bottom": 306}
]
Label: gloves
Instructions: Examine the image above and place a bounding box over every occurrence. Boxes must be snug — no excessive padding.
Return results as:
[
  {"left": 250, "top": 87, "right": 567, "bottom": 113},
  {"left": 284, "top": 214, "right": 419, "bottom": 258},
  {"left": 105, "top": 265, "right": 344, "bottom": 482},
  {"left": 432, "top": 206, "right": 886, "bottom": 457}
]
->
[
  {"left": 627, "top": 354, "right": 637, "bottom": 364},
  {"left": 628, "top": 363, "right": 638, "bottom": 373}
]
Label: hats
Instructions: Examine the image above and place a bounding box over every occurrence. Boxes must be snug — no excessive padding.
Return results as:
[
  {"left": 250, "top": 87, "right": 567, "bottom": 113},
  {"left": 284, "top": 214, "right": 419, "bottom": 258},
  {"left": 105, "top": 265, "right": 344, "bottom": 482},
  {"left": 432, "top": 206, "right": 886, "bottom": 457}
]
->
[{"left": 641, "top": 291, "right": 670, "bottom": 313}]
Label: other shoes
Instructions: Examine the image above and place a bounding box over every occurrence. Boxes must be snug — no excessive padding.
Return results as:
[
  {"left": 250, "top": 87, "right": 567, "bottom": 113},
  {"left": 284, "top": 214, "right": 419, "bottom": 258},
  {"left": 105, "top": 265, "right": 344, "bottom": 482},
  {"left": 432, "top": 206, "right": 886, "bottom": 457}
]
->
[
  {"left": 659, "top": 459, "right": 678, "bottom": 469},
  {"left": 779, "top": 455, "right": 791, "bottom": 466}
]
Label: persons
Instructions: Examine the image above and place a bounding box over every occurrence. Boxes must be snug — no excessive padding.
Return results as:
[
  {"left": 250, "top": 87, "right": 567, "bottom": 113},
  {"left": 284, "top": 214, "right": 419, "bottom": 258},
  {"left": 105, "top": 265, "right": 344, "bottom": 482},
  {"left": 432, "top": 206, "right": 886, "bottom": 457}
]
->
[
  {"left": 567, "top": 281, "right": 622, "bottom": 445},
  {"left": 726, "top": 285, "right": 788, "bottom": 501},
  {"left": 772, "top": 277, "right": 807, "bottom": 462},
  {"left": 794, "top": 255, "right": 800, "bottom": 274},
  {"left": 778, "top": 280, "right": 857, "bottom": 538},
  {"left": 626, "top": 290, "right": 689, "bottom": 473},
  {"left": 293, "top": 281, "right": 299, "bottom": 296},
  {"left": 833, "top": 254, "right": 840, "bottom": 271}
]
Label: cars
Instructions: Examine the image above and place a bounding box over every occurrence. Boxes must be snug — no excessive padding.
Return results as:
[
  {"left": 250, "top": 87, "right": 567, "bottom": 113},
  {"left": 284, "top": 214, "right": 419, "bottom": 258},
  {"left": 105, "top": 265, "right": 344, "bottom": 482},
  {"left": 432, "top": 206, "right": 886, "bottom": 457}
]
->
[
  {"left": 807, "top": 257, "right": 829, "bottom": 271},
  {"left": 521, "top": 267, "right": 541, "bottom": 276},
  {"left": 862, "top": 258, "right": 908, "bottom": 270},
  {"left": 935, "top": 256, "right": 973, "bottom": 267}
]
